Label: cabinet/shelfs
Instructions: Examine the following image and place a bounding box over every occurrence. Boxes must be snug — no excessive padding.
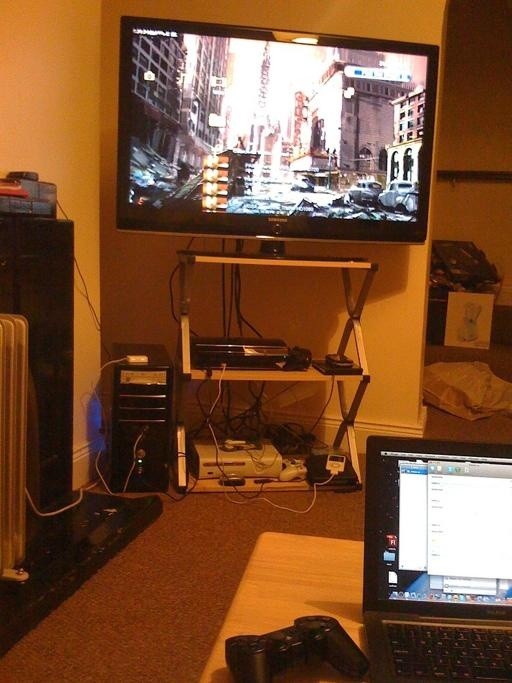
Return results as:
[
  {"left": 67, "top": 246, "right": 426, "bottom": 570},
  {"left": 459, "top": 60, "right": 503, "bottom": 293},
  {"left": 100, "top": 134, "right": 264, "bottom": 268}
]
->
[{"left": 169, "top": 249, "right": 379, "bottom": 489}]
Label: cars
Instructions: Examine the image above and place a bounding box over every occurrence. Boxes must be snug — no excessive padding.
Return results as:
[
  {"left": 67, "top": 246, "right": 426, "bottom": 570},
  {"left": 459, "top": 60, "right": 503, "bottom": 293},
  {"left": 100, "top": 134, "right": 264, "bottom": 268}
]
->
[
  {"left": 291, "top": 176, "right": 315, "bottom": 192},
  {"left": 349, "top": 179, "right": 383, "bottom": 205},
  {"left": 378, "top": 178, "right": 417, "bottom": 214}
]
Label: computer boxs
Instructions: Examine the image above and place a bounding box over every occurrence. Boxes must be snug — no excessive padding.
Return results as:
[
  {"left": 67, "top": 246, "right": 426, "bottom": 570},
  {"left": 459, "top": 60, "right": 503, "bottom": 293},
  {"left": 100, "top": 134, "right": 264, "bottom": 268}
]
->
[{"left": 110, "top": 343, "right": 174, "bottom": 492}]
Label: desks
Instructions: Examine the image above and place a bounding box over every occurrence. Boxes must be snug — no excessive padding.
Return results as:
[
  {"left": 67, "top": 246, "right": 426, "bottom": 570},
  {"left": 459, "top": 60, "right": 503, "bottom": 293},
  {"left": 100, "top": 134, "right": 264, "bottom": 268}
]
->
[{"left": 197, "top": 531, "right": 384, "bottom": 683}]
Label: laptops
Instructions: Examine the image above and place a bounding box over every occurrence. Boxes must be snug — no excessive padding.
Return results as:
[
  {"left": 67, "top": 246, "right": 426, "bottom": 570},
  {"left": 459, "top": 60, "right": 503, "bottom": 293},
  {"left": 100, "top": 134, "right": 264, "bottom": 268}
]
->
[{"left": 363, "top": 435, "right": 512, "bottom": 683}]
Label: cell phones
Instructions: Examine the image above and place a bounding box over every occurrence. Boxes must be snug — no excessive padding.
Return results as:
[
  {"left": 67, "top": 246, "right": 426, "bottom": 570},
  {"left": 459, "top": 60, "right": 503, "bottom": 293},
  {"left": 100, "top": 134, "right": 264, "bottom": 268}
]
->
[{"left": 326, "top": 354, "right": 353, "bottom": 368}]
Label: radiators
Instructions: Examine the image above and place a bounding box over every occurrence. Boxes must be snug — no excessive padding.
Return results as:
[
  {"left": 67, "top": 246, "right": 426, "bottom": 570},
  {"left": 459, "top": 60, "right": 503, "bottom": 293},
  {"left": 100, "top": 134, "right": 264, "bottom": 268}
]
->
[{"left": 0, "top": 312, "right": 30, "bottom": 586}]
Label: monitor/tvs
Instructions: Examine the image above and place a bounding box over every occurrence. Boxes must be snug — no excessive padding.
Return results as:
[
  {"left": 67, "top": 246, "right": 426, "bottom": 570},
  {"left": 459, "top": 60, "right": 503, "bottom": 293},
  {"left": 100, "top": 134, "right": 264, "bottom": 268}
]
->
[{"left": 115, "top": 16, "right": 439, "bottom": 263}]
